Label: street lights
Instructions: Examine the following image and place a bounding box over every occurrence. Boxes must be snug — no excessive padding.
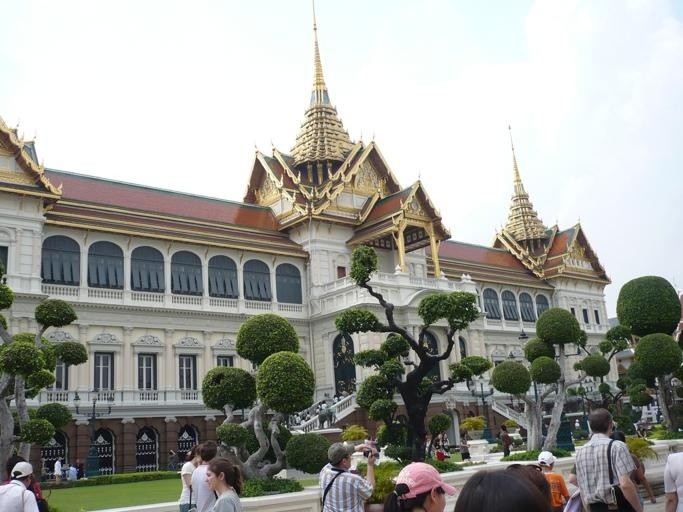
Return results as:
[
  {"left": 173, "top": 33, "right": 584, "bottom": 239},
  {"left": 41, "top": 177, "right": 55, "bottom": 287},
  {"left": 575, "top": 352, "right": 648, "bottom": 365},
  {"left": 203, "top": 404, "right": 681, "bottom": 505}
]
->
[
  {"left": 507, "top": 327, "right": 561, "bottom": 448},
  {"left": 468, "top": 373, "right": 497, "bottom": 443},
  {"left": 68, "top": 389, "right": 114, "bottom": 476},
  {"left": 652, "top": 375, "right": 662, "bottom": 421}
]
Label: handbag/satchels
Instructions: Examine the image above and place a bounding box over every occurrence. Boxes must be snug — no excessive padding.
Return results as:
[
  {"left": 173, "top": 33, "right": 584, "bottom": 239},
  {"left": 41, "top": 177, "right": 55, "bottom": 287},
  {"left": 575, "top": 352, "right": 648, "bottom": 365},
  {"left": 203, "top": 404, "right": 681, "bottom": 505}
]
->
[{"left": 590, "top": 482, "right": 636, "bottom": 512}]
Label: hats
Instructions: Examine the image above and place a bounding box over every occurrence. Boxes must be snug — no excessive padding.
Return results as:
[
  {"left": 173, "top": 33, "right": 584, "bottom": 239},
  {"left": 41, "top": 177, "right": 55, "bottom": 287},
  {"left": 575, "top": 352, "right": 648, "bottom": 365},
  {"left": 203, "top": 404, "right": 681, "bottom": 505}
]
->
[
  {"left": 327, "top": 443, "right": 355, "bottom": 466},
  {"left": 396, "top": 463, "right": 457, "bottom": 499},
  {"left": 11, "top": 462, "right": 33, "bottom": 479},
  {"left": 538, "top": 451, "right": 558, "bottom": 467}
]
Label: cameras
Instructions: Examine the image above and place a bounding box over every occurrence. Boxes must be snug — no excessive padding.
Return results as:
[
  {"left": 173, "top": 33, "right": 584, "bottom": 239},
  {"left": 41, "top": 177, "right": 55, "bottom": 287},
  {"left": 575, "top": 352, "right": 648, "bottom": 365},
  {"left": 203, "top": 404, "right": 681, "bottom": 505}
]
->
[{"left": 364, "top": 449, "right": 371, "bottom": 456}]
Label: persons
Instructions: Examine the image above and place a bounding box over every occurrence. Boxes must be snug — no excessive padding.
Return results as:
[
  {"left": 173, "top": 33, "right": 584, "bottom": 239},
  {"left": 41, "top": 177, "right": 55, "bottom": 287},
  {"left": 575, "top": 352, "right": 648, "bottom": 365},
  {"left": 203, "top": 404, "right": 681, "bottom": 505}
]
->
[
  {"left": 74, "top": 457, "right": 83, "bottom": 479},
  {"left": 178, "top": 444, "right": 202, "bottom": 512},
  {"left": 0, "top": 461, "right": 40, "bottom": 512},
  {"left": 53, "top": 455, "right": 62, "bottom": 482},
  {"left": 2, "top": 456, "right": 48, "bottom": 512},
  {"left": 65, "top": 464, "right": 78, "bottom": 481},
  {"left": 191, "top": 441, "right": 219, "bottom": 512},
  {"left": 203, "top": 457, "right": 244, "bottom": 512}
]
